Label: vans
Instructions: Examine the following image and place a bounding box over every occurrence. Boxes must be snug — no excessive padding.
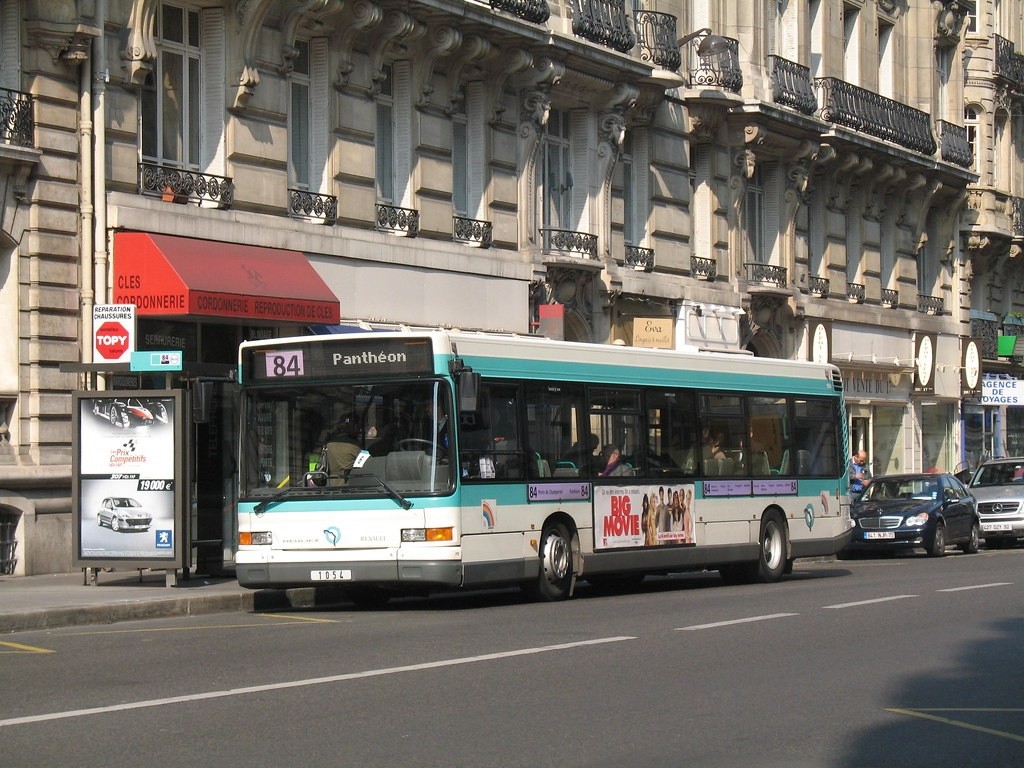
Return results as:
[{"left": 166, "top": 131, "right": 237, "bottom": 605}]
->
[{"left": 966, "top": 457, "right": 1024, "bottom": 549}]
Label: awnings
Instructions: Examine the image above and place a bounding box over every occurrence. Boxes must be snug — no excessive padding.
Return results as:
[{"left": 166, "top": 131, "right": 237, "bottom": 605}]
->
[{"left": 114, "top": 231, "right": 341, "bottom": 323}]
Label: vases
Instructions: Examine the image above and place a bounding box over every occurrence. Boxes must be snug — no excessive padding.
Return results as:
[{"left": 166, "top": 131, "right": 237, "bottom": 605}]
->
[
  {"left": 970, "top": 309, "right": 998, "bottom": 321},
  {"left": 998, "top": 335, "right": 1017, "bottom": 357}
]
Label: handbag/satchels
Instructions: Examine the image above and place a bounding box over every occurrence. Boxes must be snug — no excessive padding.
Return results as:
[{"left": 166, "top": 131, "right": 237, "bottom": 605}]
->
[{"left": 312, "top": 438, "right": 329, "bottom": 486}]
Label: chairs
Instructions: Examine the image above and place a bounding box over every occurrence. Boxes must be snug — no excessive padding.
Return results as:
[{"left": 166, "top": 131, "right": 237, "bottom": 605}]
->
[
  {"left": 551, "top": 461, "right": 580, "bottom": 477},
  {"left": 763, "top": 451, "right": 772, "bottom": 475},
  {"left": 608, "top": 462, "right": 636, "bottom": 477},
  {"left": 718, "top": 458, "right": 736, "bottom": 476},
  {"left": 529, "top": 450, "right": 551, "bottom": 478},
  {"left": 771, "top": 450, "right": 814, "bottom": 476},
  {"left": 684, "top": 457, "right": 719, "bottom": 477}
]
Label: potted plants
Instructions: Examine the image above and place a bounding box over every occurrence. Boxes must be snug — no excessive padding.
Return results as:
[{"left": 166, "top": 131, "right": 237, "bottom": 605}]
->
[{"left": 1005, "top": 312, "right": 1024, "bottom": 325}]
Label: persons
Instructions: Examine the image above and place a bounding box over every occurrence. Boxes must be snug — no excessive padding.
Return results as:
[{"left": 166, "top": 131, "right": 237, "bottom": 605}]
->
[
  {"left": 562, "top": 433, "right": 622, "bottom": 477},
  {"left": 426, "top": 395, "right": 464, "bottom": 451},
  {"left": 641, "top": 487, "right": 693, "bottom": 546},
  {"left": 848, "top": 450, "right": 872, "bottom": 503},
  {"left": 682, "top": 425, "right": 728, "bottom": 471},
  {"left": 324, "top": 413, "right": 363, "bottom": 486}
]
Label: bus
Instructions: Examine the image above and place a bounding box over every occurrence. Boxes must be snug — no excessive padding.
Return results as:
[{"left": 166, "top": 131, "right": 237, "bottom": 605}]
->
[{"left": 193, "top": 330, "right": 852, "bottom": 603}]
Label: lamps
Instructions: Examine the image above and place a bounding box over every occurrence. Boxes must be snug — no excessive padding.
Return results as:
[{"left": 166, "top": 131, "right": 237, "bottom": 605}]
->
[
  {"left": 961, "top": 366, "right": 978, "bottom": 377},
  {"left": 832, "top": 352, "right": 854, "bottom": 362},
  {"left": 880, "top": 356, "right": 900, "bottom": 367},
  {"left": 935, "top": 362, "right": 940, "bottom": 373},
  {"left": 677, "top": 27, "right": 731, "bottom": 63},
  {"left": 853, "top": 353, "right": 879, "bottom": 364},
  {"left": 940, "top": 363, "right": 962, "bottom": 375},
  {"left": 899, "top": 358, "right": 921, "bottom": 366}
]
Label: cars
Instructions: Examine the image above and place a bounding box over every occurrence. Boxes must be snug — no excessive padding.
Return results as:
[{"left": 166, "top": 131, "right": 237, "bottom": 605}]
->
[
  {"left": 837, "top": 473, "right": 980, "bottom": 559},
  {"left": 97, "top": 496, "right": 152, "bottom": 531}
]
